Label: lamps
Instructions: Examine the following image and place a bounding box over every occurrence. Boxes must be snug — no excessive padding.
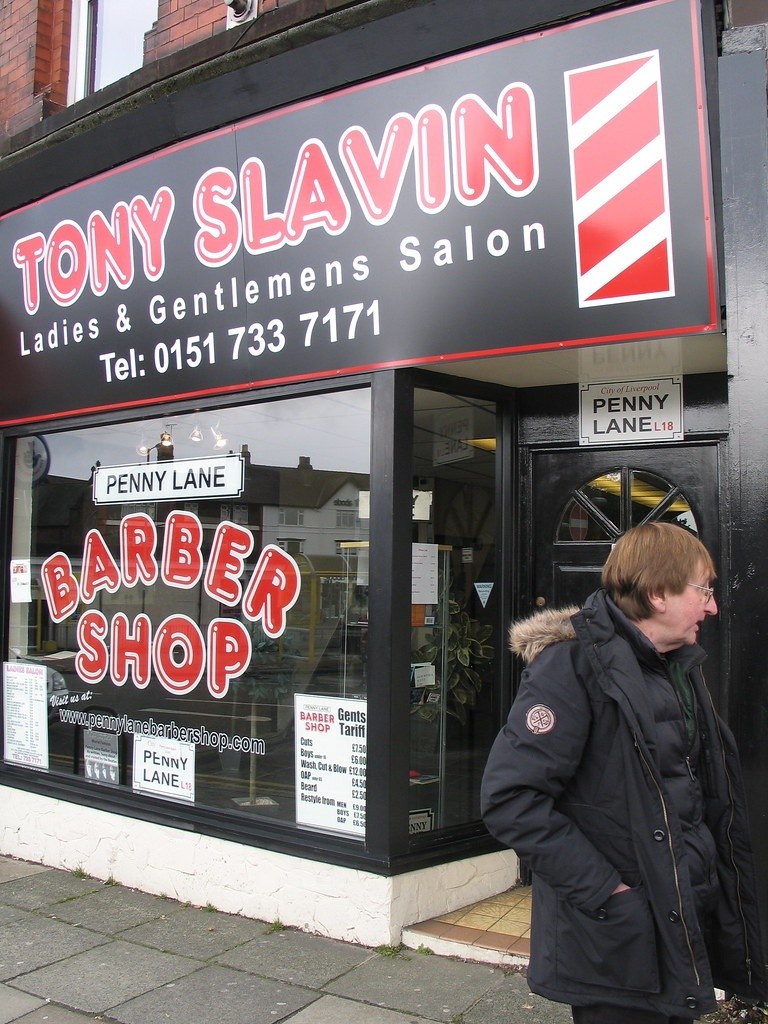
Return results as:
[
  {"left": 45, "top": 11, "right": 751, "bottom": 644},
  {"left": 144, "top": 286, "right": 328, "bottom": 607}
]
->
[
  {"left": 161, "top": 434, "right": 174, "bottom": 446},
  {"left": 136, "top": 433, "right": 152, "bottom": 457},
  {"left": 211, "top": 425, "right": 229, "bottom": 451},
  {"left": 189, "top": 423, "right": 204, "bottom": 442}
]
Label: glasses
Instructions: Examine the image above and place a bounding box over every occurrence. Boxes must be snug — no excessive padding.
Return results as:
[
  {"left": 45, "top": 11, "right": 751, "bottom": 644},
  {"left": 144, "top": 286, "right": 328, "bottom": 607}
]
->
[{"left": 687, "top": 583, "right": 714, "bottom": 603}]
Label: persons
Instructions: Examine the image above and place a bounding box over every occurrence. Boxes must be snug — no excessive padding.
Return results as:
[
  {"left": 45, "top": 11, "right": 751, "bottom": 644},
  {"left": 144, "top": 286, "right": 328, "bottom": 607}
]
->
[{"left": 483, "top": 524, "right": 768, "bottom": 1024}]
